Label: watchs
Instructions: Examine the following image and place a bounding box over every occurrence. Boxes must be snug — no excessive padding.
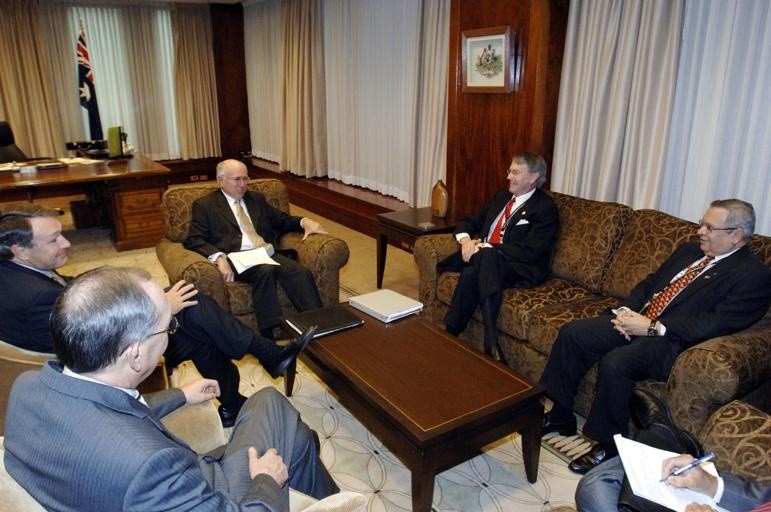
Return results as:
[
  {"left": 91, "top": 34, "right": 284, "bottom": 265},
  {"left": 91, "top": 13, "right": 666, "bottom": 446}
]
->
[{"left": 647, "top": 319, "right": 658, "bottom": 336}]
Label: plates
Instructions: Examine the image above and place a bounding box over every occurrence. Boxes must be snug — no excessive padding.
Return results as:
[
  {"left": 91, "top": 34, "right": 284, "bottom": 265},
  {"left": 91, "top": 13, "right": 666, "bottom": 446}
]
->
[{"left": 0, "top": 163, "right": 15, "bottom": 172}]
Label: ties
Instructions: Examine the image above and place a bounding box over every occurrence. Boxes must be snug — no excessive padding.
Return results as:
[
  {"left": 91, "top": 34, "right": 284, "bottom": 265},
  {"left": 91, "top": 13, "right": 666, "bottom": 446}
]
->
[
  {"left": 489, "top": 198, "right": 515, "bottom": 245},
  {"left": 643, "top": 255, "right": 714, "bottom": 321},
  {"left": 235, "top": 198, "right": 267, "bottom": 248}
]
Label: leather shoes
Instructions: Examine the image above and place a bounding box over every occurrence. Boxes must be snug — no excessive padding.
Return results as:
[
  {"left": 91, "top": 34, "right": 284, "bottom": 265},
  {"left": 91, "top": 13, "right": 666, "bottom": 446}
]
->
[
  {"left": 630, "top": 387, "right": 704, "bottom": 458},
  {"left": 216, "top": 396, "right": 248, "bottom": 428},
  {"left": 517, "top": 413, "right": 577, "bottom": 436},
  {"left": 569, "top": 442, "right": 609, "bottom": 473},
  {"left": 483, "top": 340, "right": 508, "bottom": 366},
  {"left": 261, "top": 326, "right": 317, "bottom": 379}
]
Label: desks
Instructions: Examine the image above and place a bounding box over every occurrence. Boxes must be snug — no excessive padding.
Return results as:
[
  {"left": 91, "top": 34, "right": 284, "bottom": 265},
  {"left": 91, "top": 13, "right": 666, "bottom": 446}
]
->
[
  {"left": 0, "top": 154, "right": 173, "bottom": 252},
  {"left": 376, "top": 207, "right": 465, "bottom": 289}
]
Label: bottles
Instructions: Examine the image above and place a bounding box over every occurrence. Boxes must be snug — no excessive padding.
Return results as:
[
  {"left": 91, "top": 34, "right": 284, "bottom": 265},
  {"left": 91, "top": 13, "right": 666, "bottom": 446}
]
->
[
  {"left": 430, "top": 180, "right": 449, "bottom": 217},
  {"left": 10, "top": 161, "right": 18, "bottom": 171}
]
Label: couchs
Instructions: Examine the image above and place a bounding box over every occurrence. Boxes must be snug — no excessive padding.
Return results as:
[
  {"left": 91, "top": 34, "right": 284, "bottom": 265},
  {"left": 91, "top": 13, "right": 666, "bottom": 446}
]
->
[{"left": 414, "top": 189, "right": 771, "bottom": 438}]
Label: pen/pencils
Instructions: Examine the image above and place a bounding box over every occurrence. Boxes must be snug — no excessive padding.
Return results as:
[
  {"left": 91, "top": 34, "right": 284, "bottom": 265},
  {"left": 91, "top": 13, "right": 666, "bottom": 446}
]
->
[{"left": 659, "top": 452, "right": 716, "bottom": 483}]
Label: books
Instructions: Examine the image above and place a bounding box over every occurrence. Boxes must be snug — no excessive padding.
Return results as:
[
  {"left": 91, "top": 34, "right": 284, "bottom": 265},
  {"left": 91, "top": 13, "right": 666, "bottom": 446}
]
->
[
  {"left": 284, "top": 306, "right": 365, "bottom": 340},
  {"left": 612, "top": 432, "right": 729, "bottom": 512}
]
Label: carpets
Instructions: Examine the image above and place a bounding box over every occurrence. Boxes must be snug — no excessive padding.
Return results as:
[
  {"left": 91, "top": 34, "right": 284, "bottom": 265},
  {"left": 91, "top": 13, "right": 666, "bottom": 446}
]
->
[{"left": 55, "top": 212, "right": 599, "bottom": 512}]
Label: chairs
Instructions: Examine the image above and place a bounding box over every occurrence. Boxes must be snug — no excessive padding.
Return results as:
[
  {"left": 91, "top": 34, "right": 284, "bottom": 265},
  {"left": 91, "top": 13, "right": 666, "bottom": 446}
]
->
[
  {"left": 156, "top": 178, "right": 349, "bottom": 340},
  {"left": 551, "top": 400, "right": 771, "bottom": 512},
  {"left": 0, "top": 400, "right": 368, "bottom": 512},
  {"left": 0, "top": 340, "right": 168, "bottom": 436},
  {"left": 0, "top": 122, "right": 28, "bottom": 163}
]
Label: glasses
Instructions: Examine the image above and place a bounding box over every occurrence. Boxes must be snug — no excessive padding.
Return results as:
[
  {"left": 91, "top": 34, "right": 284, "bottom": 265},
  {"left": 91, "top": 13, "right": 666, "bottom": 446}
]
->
[
  {"left": 121, "top": 316, "right": 180, "bottom": 355},
  {"left": 695, "top": 219, "right": 736, "bottom": 232}
]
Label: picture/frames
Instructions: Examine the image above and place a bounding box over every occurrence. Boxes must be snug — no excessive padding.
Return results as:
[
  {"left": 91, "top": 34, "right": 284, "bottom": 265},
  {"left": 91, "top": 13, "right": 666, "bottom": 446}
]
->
[{"left": 458, "top": 25, "right": 515, "bottom": 95}]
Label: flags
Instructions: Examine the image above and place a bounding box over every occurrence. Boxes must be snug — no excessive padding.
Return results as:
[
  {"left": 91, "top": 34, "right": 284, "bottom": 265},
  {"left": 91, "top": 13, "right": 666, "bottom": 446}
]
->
[{"left": 75, "top": 32, "right": 103, "bottom": 141}]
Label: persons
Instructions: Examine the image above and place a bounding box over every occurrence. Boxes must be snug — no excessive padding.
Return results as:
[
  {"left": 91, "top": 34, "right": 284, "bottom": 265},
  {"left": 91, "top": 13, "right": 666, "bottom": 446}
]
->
[
  {"left": 1, "top": 206, "right": 319, "bottom": 429},
  {"left": 575, "top": 388, "right": 771, "bottom": 511},
  {"left": 517, "top": 198, "right": 771, "bottom": 475},
  {"left": 4, "top": 267, "right": 340, "bottom": 512},
  {"left": 184, "top": 158, "right": 323, "bottom": 338},
  {"left": 437, "top": 149, "right": 561, "bottom": 366}
]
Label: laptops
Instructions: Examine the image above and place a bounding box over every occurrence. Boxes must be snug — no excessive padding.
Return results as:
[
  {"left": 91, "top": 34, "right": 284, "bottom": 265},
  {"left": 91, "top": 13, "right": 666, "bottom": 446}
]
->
[
  {"left": 24, "top": 158, "right": 69, "bottom": 171},
  {"left": 348, "top": 288, "right": 424, "bottom": 322}
]
